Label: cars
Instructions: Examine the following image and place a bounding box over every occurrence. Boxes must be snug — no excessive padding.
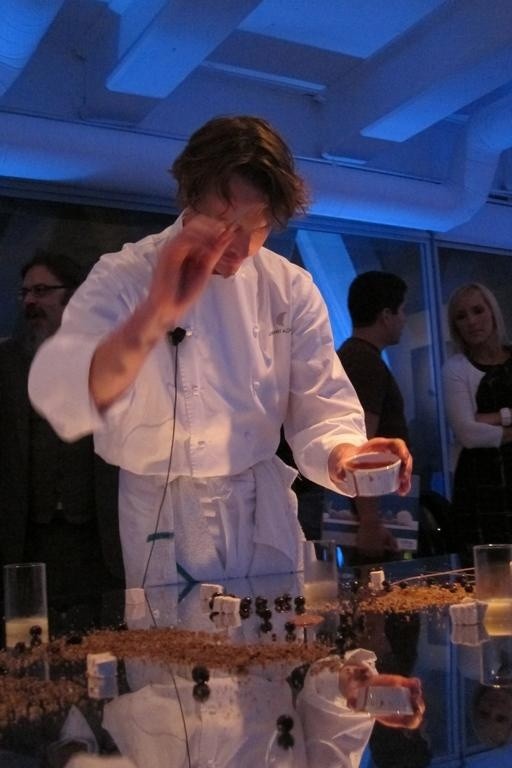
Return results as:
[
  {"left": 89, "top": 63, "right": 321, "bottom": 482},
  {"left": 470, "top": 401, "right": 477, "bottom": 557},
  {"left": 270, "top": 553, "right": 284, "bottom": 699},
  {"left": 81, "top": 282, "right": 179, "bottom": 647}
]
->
[
  {"left": 0, "top": 562, "right": 50, "bottom": 666},
  {"left": 473, "top": 543, "right": 512, "bottom": 636},
  {"left": 299, "top": 538, "right": 337, "bottom": 591}
]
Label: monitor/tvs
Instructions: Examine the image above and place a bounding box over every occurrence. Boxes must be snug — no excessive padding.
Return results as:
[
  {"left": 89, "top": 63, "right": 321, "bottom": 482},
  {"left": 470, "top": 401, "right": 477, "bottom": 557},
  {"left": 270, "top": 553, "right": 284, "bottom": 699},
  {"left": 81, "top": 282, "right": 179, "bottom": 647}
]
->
[{"left": 321, "top": 474, "right": 421, "bottom": 550}]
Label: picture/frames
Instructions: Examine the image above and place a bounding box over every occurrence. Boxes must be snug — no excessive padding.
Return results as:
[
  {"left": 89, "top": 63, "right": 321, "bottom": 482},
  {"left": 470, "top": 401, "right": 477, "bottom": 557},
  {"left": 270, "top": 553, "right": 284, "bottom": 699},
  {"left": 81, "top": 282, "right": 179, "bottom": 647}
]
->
[{"left": 4, "top": 552, "right": 511, "bottom": 766}]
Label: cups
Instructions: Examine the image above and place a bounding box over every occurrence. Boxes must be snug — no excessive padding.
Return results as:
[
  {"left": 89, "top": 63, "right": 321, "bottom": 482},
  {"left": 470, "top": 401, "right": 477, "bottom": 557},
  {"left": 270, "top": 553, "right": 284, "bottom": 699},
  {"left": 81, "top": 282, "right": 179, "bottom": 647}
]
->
[{"left": 14, "top": 283, "right": 66, "bottom": 302}]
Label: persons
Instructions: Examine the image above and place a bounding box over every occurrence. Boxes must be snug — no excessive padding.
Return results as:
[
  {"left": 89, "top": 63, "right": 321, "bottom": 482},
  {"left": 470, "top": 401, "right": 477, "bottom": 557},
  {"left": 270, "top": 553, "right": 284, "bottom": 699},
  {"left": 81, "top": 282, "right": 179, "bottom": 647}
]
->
[
  {"left": 1, "top": 260, "right": 120, "bottom": 630},
  {"left": 27, "top": 115, "right": 414, "bottom": 588},
  {"left": 442, "top": 281, "right": 512, "bottom": 580},
  {"left": 321, "top": 271, "right": 419, "bottom": 564},
  {"left": 47, "top": 588, "right": 425, "bottom": 768}
]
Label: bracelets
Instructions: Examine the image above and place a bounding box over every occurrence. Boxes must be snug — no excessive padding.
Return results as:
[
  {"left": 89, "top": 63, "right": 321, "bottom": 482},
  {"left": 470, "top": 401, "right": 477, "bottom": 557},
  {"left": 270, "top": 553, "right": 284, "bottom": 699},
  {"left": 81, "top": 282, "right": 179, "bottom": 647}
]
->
[
  {"left": 355, "top": 686, "right": 414, "bottom": 719},
  {"left": 341, "top": 451, "right": 402, "bottom": 497}
]
